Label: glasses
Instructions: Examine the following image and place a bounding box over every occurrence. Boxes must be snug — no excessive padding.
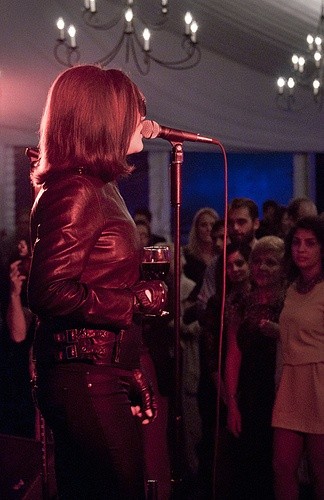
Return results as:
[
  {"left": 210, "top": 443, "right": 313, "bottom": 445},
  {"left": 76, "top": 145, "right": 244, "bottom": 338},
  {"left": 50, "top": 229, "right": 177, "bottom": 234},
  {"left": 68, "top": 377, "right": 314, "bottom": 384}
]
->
[{"left": 138, "top": 99, "right": 147, "bottom": 117}]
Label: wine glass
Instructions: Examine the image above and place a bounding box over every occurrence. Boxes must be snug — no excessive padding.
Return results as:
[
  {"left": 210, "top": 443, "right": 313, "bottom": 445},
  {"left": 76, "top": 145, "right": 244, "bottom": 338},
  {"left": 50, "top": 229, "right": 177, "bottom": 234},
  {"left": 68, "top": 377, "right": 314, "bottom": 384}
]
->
[{"left": 140, "top": 246, "right": 172, "bottom": 317}]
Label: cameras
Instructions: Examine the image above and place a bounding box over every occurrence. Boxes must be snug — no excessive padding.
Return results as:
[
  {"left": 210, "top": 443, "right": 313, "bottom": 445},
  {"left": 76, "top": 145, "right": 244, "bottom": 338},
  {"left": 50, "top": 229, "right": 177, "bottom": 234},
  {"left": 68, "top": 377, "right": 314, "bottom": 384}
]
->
[{"left": 18, "top": 259, "right": 31, "bottom": 275}]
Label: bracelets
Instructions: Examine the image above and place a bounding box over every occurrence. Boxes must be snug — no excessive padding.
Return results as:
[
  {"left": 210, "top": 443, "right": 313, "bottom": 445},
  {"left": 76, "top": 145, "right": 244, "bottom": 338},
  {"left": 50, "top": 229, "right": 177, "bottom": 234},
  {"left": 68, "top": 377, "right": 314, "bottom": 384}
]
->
[{"left": 225, "top": 393, "right": 238, "bottom": 400}]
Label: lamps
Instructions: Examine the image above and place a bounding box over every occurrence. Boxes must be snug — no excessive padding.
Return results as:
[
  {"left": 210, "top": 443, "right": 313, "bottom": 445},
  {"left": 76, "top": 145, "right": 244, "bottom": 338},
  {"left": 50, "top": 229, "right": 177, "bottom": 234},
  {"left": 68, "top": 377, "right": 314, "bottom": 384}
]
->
[{"left": 53, "top": 0, "right": 324, "bottom": 113}]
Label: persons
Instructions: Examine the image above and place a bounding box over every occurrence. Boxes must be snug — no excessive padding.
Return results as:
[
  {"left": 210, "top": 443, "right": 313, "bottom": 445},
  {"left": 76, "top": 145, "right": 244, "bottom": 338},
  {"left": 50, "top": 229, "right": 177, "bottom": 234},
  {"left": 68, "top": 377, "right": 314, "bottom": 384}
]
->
[
  {"left": 26, "top": 63, "right": 171, "bottom": 500},
  {"left": 0, "top": 195, "right": 324, "bottom": 500}
]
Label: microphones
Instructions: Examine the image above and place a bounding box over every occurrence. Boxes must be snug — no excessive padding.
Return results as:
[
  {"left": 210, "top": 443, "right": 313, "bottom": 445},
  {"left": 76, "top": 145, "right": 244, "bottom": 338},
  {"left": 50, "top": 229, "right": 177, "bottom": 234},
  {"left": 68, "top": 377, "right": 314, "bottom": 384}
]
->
[{"left": 142, "top": 120, "right": 220, "bottom": 145}]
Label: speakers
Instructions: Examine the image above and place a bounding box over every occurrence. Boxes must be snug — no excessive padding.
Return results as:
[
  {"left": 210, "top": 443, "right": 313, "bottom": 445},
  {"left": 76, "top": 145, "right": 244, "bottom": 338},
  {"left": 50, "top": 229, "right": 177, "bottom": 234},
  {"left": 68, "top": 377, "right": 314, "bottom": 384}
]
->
[{"left": 0, "top": 434, "right": 43, "bottom": 500}]
